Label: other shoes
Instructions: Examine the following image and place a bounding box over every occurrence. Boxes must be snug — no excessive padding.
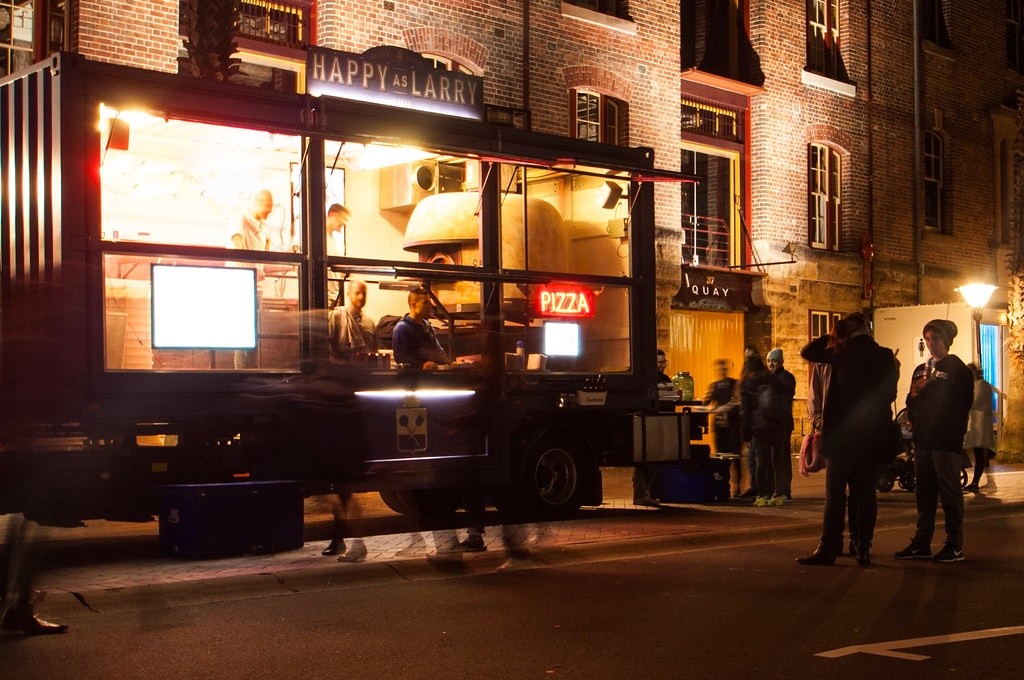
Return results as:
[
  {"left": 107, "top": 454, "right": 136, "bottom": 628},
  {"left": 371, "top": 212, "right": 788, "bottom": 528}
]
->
[
  {"left": 794, "top": 543, "right": 836, "bottom": 562},
  {"left": 752, "top": 495, "right": 766, "bottom": 507},
  {"left": 848, "top": 537, "right": 872, "bottom": 564},
  {"left": 634, "top": 497, "right": 660, "bottom": 506},
  {"left": 337, "top": 545, "right": 368, "bottom": 561},
  {"left": 1, "top": 613, "right": 68, "bottom": 634},
  {"left": 963, "top": 484, "right": 980, "bottom": 491},
  {"left": 766, "top": 493, "right": 784, "bottom": 505},
  {"left": 456, "top": 534, "right": 484, "bottom": 548},
  {"left": 752, "top": 490, "right": 792, "bottom": 503},
  {"left": 321, "top": 538, "right": 346, "bottom": 554}
]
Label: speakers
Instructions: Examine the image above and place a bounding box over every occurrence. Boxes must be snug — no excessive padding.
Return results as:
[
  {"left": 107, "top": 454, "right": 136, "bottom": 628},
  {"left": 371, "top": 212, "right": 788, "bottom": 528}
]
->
[
  {"left": 595, "top": 181, "right": 623, "bottom": 209},
  {"left": 109, "top": 118, "right": 130, "bottom": 150}
]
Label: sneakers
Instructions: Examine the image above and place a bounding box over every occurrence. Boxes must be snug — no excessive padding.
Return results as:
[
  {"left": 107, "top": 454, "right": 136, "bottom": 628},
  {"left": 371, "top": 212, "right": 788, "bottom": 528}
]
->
[
  {"left": 894, "top": 541, "right": 932, "bottom": 558},
  {"left": 932, "top": 542, "right": 965, "bottom": 562}
]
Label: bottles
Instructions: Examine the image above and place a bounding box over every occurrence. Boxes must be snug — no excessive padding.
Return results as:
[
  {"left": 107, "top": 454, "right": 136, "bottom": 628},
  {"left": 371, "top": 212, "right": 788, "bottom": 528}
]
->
[
  {"left": 672, "top": 371, "right": 694, "bottom": 402},
  {"left": 516, "top": 340, "right": 525, "bottom": 371}
]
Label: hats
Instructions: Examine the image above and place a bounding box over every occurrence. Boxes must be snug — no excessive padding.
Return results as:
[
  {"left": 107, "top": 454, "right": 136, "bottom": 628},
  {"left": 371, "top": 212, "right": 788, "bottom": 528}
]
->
[
  {"left": 923, "top": 319, "right": 957, "bottom": 347},
  {"left": 766, "top": 348, "right": 783, "bottom": 363}
]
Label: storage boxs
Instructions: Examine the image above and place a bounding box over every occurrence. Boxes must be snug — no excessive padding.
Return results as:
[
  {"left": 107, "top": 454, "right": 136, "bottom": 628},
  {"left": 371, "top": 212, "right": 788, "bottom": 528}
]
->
[
  {"left": 155, "top": 479, "right": 306, "bottom": 560},
  {"left": 633, "top": 461, "right": 731, "bottom": 503}
]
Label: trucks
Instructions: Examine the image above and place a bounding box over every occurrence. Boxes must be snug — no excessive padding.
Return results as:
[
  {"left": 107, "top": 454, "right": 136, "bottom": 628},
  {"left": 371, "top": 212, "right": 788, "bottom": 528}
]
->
[{"left": 0, "top": 50, "right": 706, "bottom": 520}]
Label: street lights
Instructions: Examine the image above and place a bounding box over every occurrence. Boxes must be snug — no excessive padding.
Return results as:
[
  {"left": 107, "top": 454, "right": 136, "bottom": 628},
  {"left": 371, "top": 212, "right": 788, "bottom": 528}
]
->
[{"left": 959, "top": 281, "right": 1000, "bottom": 369}]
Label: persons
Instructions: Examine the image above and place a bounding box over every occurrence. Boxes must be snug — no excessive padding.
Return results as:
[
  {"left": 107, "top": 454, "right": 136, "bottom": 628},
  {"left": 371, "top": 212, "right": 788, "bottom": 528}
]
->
[
  {"left": 320, "top": 368, "right": 559, "bottom": 569},
  {"left": 230, "top": 191, "right": 275, "bottom": 369},
  {"left": 1, "top": 274, "right": 97, "bottom": 634},
  {"left": 325, "top": 203, "right": 351, "bottom": 311},
  {"left": 657, "top": 348, "right": 675, "bottom": 411},
  {"left": 392, "top": 288, "right": 450, "bottom": 371},
  {"left": 797, "top": 312, "right": 994, "bottom": 567},
  {"left": 702, "top": 347, "right": 796, "bottom": 506},
  {"left": 328, "top": 281, "right": 379, "bottom": 371}
]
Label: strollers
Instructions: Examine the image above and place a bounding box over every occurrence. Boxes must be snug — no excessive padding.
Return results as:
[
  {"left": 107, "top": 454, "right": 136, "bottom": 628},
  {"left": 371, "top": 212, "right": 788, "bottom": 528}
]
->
[{"left": 878, "top": 408, "right": 968, "bottom": 493}]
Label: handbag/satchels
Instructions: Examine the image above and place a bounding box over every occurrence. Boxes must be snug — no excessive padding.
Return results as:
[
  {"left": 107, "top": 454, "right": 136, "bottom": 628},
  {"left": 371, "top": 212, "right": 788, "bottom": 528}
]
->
[{"left": 892, "top": 422, "right": 905, "bottom": 455}]
